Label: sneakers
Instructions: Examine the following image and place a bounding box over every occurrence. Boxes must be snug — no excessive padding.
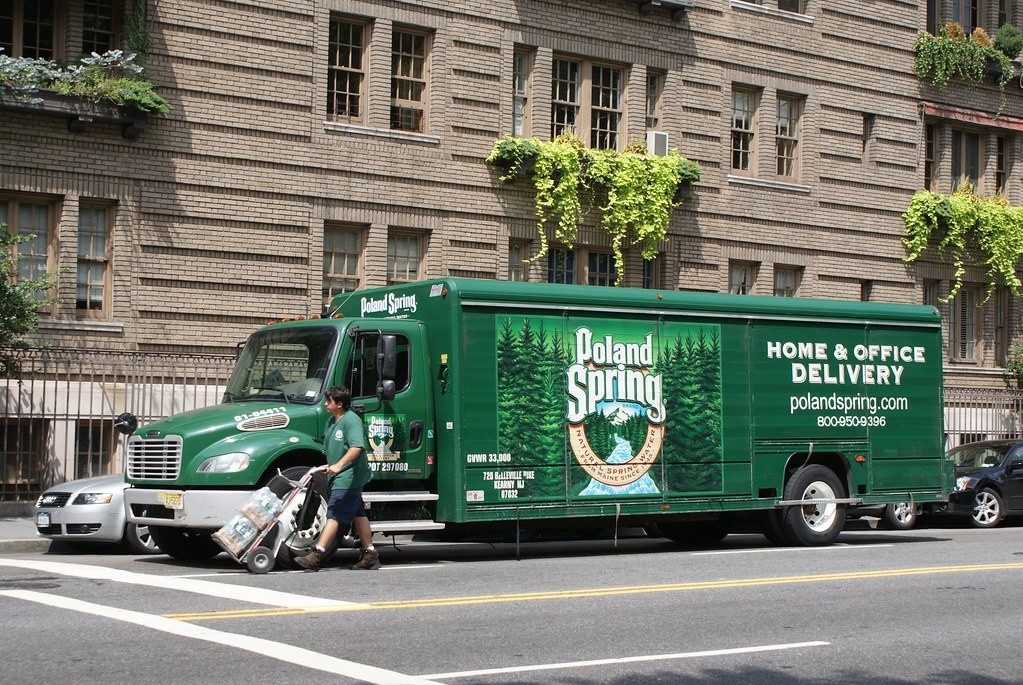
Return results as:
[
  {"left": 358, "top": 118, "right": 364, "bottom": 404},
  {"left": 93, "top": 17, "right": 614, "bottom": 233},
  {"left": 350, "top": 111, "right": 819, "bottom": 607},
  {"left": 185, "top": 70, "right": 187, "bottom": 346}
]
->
[
  {"left": 348, "top": 548, "right": 382, "bottom": 570},
  {"left": 293, "top": 545, "right": 329, "bottom": 571}
]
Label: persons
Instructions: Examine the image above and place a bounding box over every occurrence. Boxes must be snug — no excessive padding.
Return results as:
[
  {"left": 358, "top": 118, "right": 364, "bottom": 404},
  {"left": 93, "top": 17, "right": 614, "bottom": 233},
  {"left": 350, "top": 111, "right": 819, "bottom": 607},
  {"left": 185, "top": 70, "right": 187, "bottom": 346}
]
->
[{"left": 293, "top": 385, "right": 383, "bottom": 571}]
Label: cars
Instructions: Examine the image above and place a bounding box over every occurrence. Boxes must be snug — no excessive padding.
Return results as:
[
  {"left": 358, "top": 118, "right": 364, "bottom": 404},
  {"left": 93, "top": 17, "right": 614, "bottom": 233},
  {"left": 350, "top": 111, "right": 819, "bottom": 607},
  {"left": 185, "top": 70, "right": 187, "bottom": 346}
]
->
[
  {"left": 33, "top": 474, "right": 164, "bottom": 555},
  {"left": 915, "top": 439, "right": 1023, "bottom": 528}
]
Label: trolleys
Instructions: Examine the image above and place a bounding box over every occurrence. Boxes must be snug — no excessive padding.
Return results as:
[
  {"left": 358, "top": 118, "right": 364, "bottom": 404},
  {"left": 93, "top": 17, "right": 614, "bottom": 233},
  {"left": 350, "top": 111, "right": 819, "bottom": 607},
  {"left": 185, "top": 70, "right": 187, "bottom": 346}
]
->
[{"left": 211, "top": 465, "right": 332, "bottom": 576}]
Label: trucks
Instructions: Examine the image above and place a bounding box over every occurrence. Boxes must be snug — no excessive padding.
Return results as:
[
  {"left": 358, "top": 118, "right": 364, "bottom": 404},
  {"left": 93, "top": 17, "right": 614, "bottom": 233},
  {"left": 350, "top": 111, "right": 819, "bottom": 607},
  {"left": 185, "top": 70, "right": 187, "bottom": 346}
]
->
[{"left": 112, "top": 278, "right": 956, "bottom": 575}]
